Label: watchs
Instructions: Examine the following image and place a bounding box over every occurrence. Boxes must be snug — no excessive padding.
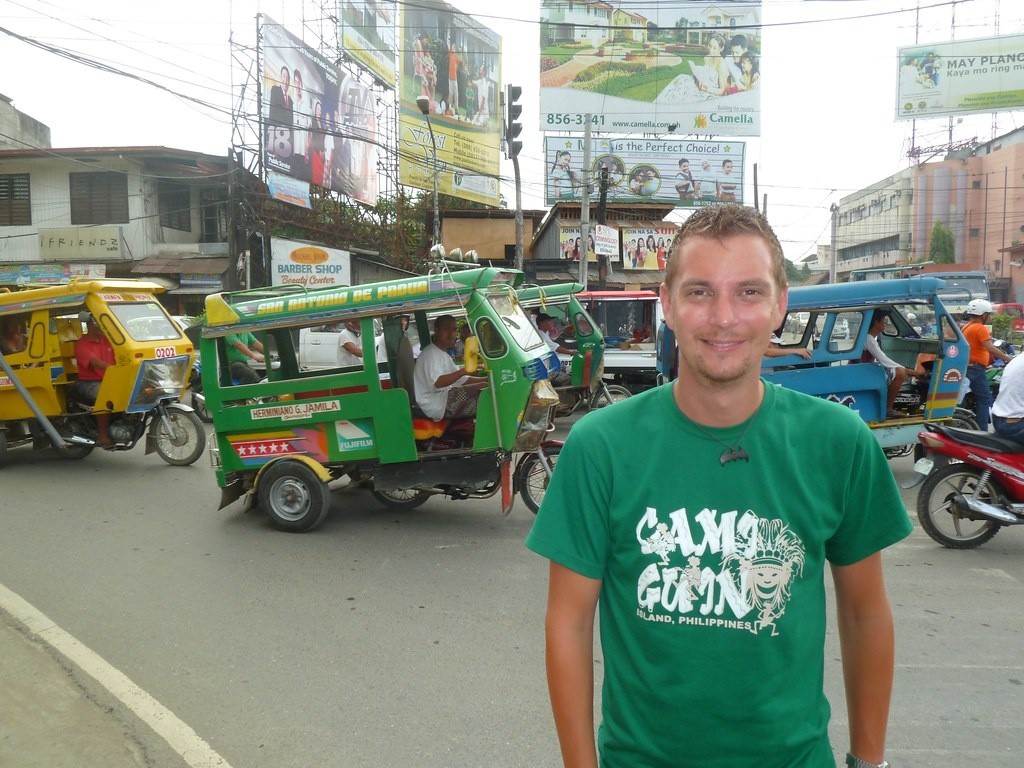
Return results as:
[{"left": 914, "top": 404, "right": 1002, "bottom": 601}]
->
[{"left": 845, "top": 752, "right": 892, "bottom": 768}]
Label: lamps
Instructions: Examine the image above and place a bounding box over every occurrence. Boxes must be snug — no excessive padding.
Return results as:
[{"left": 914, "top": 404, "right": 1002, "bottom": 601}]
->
[{"left": 668, "top": 125, "right": 677, "bottom": 132}]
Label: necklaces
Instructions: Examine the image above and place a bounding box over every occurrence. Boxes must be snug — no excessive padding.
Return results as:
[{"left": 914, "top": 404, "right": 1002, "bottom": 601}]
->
[{"left": 685, "top": 409, "right": 758, "bottom": 467}]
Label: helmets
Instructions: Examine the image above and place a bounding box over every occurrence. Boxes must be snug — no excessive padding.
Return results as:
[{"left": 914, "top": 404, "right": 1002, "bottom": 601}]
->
[
  {"left": 393, "top": 314, "right": 410, "bottom": 331},
  {"left": 907, "top": 313, "right": 917, "bottom": 320},
  {"left": 344, "top": 318, "right": 361, "bottom": 334},
  {"left": 967, "top": 298, "right": 992, "bottom": 315}
]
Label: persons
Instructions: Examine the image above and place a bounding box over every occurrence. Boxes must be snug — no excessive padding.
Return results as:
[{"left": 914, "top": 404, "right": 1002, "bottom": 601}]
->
[
  {"left": 561, "top": 234, "right": 597, "bottom": 261},
  {"left": 413, "top": 32, "right": 492, "bottom": 126},
  {"left": 900, "top": 51, "right": 943, "bottom": 93},
  {"left": 335, "top": 312, "right": 578, "bottom": 421},
  {"left": 550, "top": 150, "right": 738, "bottom": 203},
  {"left": 225, "top": 331, "right": 270, "bottom": 384},
  {"left": 857, "top": 298, "right": 1012, "bottom": 432},
  {"left": 74, "top": 314, "right": 115, "bottom": 450},
  {"left": 267, "top": 66, "right": 369, "bottom": 203},
  {"left": 0, "top": 317, "right": 25, "bottom": 356},
  {"left": 623, "top": 235, "right": 672, "bottom": 270},
  {"left": 525, "top": 204, "right": 914, "bottom": 768},
  {"left": 765, "top": 332, "right": 812, "bottom": 371},
  {"left": 652, "top": 34, "right": 760, "bottom": 106},
  {"left": 992, "top": 345, "right": 1024, "bottom": 441}
]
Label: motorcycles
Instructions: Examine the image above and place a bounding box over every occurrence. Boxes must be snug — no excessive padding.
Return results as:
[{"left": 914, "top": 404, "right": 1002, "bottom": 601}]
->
[
  {"left": 125, "top": 315, "right": 218, "bottom": 425},
  {"left": 0, "top": 272, "right": 206, "bottom": 470},
  {"left": 183, "top": 243, "right": 1014, "bottom": 534},
  {"left": 900, "top": 422, "right": 1024, "bottom": 551}
]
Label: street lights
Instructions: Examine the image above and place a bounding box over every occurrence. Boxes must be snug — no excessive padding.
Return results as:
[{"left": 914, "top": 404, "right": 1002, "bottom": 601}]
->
[{"left": 415, "top": 95, "right": 441, "bottom": 252}]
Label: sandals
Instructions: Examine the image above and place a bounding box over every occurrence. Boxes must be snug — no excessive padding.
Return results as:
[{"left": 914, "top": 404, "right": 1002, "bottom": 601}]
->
[{"left": 885, "top": 409, "right": 906, "bottom": 418}]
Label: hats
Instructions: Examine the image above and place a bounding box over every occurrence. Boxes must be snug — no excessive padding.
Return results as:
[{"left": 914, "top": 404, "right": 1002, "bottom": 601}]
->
[{"left": 535, "top": 312, "right": 556, "bottom": 324}]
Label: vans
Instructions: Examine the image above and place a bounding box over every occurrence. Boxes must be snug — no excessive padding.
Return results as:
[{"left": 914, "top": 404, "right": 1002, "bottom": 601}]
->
[{"left": 992, "top": 303, "right": 1024, "bottom": 334}]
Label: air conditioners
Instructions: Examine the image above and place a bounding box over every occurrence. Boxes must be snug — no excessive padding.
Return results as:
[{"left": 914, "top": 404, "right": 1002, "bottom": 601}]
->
[
  {"left": 908, "top": 190, "right": 913, "bottom": 194},
  {"left": 906, "top": 244, "right": 911, "bottom": 250},
  {"left": 895, "top": 190, "right": 901, "bottom": 196}
]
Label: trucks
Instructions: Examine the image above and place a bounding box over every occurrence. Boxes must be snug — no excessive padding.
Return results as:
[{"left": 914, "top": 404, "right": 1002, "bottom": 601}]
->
[{"left": 909, "top": 272, "right": 992, "bottom": 343}]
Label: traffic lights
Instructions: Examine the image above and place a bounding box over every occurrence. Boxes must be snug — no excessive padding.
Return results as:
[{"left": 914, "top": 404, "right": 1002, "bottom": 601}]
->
[{"left": 506, "top": 84, "right": 524, "bottom": 163}]
